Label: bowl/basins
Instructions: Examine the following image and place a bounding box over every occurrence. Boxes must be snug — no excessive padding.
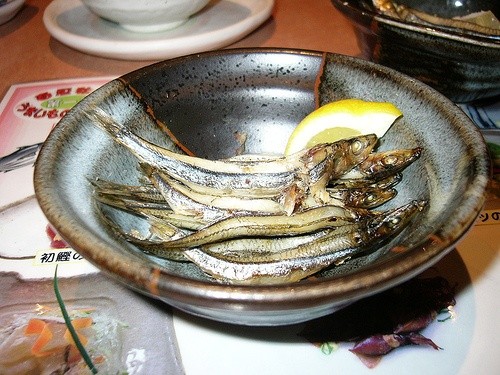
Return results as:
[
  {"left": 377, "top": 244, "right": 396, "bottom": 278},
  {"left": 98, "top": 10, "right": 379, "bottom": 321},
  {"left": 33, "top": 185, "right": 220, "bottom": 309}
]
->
[
  {"left": 33, "top": 47, "right": 491, "bottom": 325},
  {"left": 331, "top": 0, "right": 500, "bottom": 101},
  {"left": 81, "top": 0, "right": 210, "bottom": 32}
]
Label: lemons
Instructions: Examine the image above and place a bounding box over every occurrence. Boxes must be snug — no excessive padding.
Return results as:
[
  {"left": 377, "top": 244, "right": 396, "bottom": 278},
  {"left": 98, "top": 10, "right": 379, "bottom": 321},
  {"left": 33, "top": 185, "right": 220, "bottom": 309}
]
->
[{"left": 284, "top": 99, "right": 402, "bottom": 157}]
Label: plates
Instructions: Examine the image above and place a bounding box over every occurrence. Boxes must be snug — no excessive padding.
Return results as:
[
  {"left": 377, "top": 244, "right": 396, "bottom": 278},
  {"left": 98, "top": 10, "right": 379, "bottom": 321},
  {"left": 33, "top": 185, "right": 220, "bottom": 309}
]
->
[{"left": 42, "top": 0, "right": 275, "bottom": 60}]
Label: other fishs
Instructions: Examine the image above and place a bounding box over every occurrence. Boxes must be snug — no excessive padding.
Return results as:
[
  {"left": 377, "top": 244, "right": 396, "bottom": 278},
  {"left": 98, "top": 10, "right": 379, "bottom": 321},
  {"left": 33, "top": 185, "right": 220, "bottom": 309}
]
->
[
  {"left": 370, "top": 0, "right": 500, "bottom": 42},
  {"left": 82, "top": 102, "right": 427, "bottom": 288}
]
